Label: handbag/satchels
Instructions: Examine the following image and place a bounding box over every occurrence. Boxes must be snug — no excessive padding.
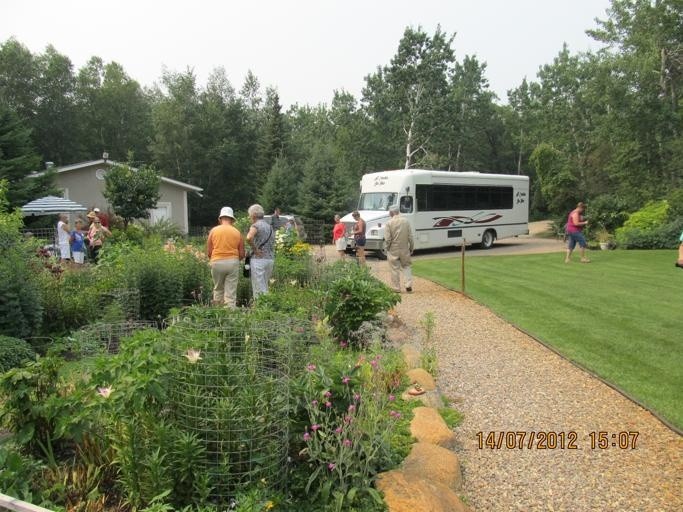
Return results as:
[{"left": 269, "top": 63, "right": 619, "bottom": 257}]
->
[{"left": 243, "top": 257, "right": 251, "bottom": 277}]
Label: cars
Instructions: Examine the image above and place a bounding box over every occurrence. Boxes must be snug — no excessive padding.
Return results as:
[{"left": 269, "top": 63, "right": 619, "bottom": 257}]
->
[{"left": 263, "top": 214, "right": 307, "bottom": 242}]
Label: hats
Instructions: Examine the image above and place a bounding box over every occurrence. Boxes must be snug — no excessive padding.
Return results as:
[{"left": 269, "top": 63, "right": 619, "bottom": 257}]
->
[
  {"left": 86, "top": 211, "right": 96, "bottom": 218},
  {"left": 94, "top": 208, "right": 100, "bottom": 212},
  {"left": 218, "top": 207, "right": 237, "bottom": 223}
]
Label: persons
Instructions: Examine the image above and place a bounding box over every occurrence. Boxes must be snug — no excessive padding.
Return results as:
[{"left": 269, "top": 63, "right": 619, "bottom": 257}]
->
[
  {"left": 57, "top": 208, "right": 111, "bottom": 266},
  {"left": 351, "top": 212, "right": 366, "bottom": 265},
  {"left": 675, "top": 233, "right": 682, "bottom": 265},
  {"left": 269, "top": 207, "right": 280, "bottom": 233},
  {"left": 563, "top": 202, "right": 591, "bottom": 262},
  {"left": 245, "top": 203, "right": 275, "bottom": 298},
  {"left": 331, "top": 214, "right": 346, "bottom": 262},
  {"left": 285, "top": 217, "right": 296, "bottom": 233},
  {"left": 381, "top": 204, "right": 415, "bottom": 296},
  {"left": 206, "top": 207, "right": 244, "bottom": 307}
]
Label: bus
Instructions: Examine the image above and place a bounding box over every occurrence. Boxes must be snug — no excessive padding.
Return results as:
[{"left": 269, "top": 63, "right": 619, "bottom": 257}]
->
[{"left": 340, "top": 169, "right": 530, "bottom": 260}]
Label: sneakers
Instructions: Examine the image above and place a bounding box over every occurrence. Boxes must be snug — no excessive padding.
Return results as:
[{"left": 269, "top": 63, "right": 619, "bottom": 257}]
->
[{"left": 406, "top": 287, "right": 412, "bottom": 291}]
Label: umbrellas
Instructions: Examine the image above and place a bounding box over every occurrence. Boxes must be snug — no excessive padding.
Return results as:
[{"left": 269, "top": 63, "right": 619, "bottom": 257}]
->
[{"left": 17, "top": 194, "right": 87, "bottom": 218}]
